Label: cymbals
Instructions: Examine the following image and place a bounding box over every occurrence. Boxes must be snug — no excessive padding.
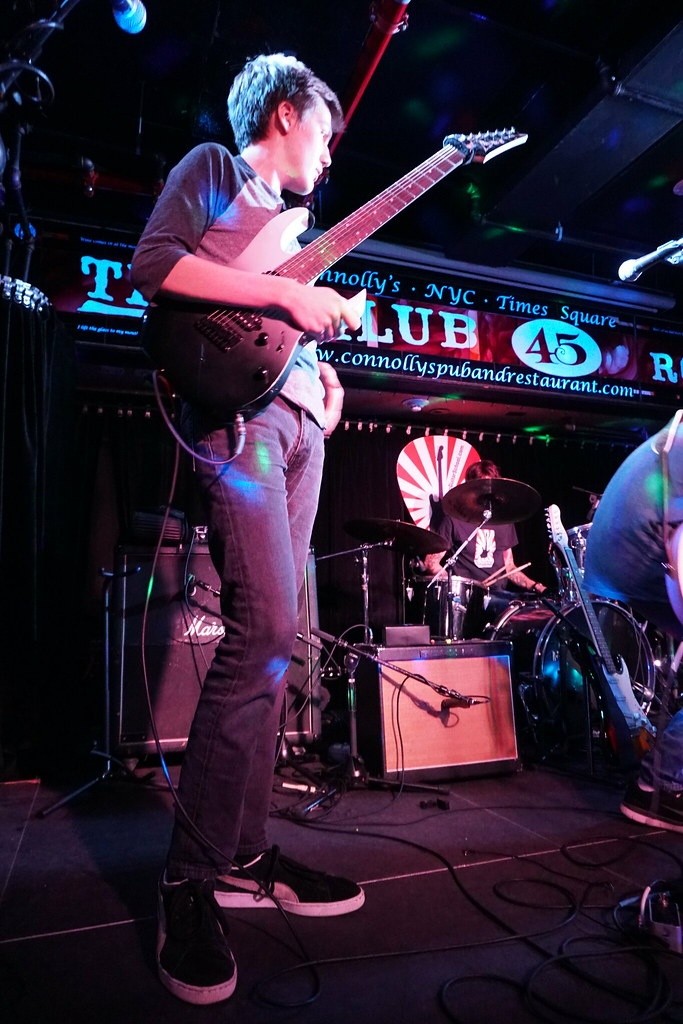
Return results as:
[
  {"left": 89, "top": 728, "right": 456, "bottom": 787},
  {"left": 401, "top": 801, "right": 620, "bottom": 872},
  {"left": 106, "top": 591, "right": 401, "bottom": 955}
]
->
[
  {"left": 442, "top": 476, "right": 542, "bottom": 526},
  {"left": 370, "top": 518, "right": 447, "bottom": 550}
]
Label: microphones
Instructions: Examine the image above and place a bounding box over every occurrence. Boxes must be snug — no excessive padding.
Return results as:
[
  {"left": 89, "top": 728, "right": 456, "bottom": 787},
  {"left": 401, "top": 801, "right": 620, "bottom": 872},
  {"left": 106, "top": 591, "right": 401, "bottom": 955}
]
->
[
  {"left": 618, "top": 235, "right": 683, "bottom": 283},
  {"left": 180, "top": 579, "right": 200, "bottom": 596},
  {"left": 109, "top": 0, "right": 148, "bottom": 35}
]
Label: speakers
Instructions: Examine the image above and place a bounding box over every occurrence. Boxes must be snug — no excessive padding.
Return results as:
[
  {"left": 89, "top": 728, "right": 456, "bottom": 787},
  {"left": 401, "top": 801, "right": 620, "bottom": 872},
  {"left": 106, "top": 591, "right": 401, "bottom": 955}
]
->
[
  {"left": 352, "top": 638, "right": 523, "bottom": 788},
  {"left": 112, "top": 543, "right": 322, "bottom": 770}
]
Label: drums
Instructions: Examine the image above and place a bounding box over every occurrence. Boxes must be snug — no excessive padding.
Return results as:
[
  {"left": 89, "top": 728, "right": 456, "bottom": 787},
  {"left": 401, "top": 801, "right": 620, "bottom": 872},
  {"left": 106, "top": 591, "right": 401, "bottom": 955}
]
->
[
  {"left": 549, "top": 521, "right": 594, "bottom": 598},
  {"left": 414, "top": 574, "right": 489, "bottom": 643},
  {"left": 489, "top": 595, "right": 654, "bottom": 757}
]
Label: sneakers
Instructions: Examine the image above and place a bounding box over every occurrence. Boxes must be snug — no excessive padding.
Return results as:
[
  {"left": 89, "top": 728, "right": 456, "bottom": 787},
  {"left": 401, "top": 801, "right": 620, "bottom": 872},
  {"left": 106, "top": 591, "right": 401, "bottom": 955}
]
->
[
  {"left": 213, "top": 844, "right": 366, "bottom": 918},
  {"left": 156, "top": 866, "right": 238, "bottom": 1005},
  {"left": 620, "top": 772, "right": 683, "bottom": 833}
]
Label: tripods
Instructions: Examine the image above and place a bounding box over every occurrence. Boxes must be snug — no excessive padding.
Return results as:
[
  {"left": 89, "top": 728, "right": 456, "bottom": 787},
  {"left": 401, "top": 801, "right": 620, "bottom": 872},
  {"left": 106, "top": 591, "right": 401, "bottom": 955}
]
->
[
  {"left": 34, "top": 549, "right": 180, "bottom": 817},
  {"left": 296, "top": 626, "right": 450, "bottom": 823}
]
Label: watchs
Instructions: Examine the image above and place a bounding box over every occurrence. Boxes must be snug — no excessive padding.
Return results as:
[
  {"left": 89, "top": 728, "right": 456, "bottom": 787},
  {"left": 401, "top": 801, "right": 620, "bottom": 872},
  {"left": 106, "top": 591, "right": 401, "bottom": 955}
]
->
[{"left": 532, "top": 582, "right": 543, "bottom": 592}]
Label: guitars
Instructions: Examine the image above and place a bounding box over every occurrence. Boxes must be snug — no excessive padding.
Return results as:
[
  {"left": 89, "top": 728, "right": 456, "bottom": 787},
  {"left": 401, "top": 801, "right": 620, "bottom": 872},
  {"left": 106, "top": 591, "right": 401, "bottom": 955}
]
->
[
  {"left": 140, "top": 123, "right": 531, "bottom": 423},
  {"left": 541, "top": 504, "right": 657, "bottom": 765}
]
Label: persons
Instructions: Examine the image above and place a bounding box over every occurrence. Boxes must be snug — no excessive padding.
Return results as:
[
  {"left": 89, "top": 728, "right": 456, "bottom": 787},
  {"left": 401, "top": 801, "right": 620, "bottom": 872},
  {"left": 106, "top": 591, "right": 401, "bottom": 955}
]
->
[
  {"left": 423, "top": 460, "right": 549, "bottom": 612},
  {"left": 127, "top": 53, "right": 364, "bottom": 1003},
  {"left": 582, "top": 408, "right": 683, "bottom": 835}
]
